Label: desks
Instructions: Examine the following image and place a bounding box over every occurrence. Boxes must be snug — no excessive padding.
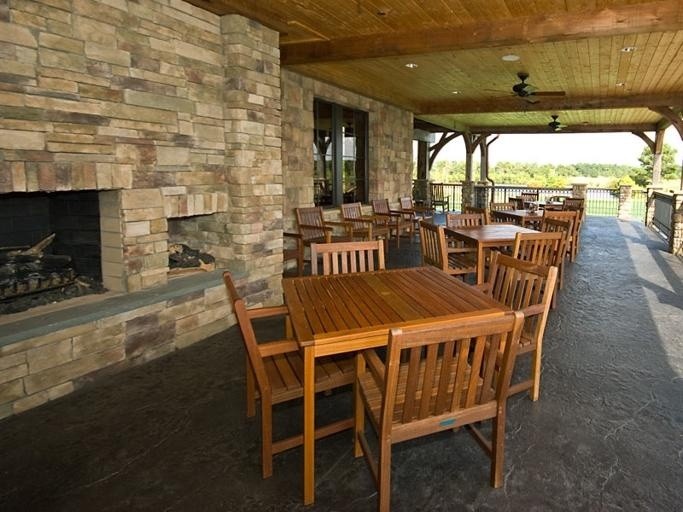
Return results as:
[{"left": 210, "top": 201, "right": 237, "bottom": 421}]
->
[{"left": 281, "top": 267, "right": 514, "bottom": 505}]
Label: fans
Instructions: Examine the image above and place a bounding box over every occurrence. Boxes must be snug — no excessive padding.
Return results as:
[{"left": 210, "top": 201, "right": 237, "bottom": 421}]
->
[
  {"left": 484, "top": 73, "right": 566, "bottom": 104},
  {"left": 549, "top": 115, "right": 567, "bottom": 131}
]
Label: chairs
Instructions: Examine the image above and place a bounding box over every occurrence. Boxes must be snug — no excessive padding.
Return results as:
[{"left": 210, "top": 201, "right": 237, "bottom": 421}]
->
[
  {"left": 468, "top": 251, "right": 558, "bottom": 402},
  {"left": 283, "top": 183, "right": 585, "bottom": 309},
  {"left": 224, "top": 270, "right": 370, "bottom": 479},
  {"left": 352, "top": 310, "right": 525, "bottom": 510}
]
